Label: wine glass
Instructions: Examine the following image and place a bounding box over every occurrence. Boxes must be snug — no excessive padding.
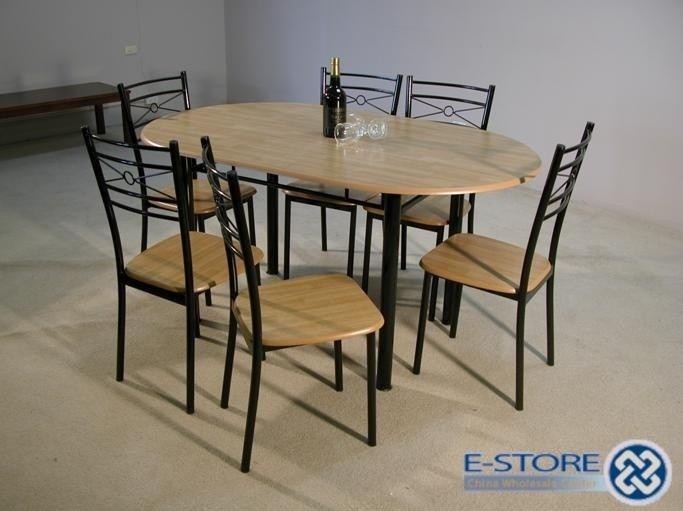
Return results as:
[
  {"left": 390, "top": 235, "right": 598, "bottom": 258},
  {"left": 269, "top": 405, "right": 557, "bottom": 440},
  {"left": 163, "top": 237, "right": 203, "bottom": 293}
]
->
[{"left": 334, "top": 112, "right": 389, "bottom": 145}]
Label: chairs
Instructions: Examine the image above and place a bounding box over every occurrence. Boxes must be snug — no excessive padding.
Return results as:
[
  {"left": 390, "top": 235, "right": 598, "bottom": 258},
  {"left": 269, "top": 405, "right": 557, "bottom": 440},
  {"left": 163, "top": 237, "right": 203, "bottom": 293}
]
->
[{"left": 80, "top": 66, "right": 597, "bottom": 477}]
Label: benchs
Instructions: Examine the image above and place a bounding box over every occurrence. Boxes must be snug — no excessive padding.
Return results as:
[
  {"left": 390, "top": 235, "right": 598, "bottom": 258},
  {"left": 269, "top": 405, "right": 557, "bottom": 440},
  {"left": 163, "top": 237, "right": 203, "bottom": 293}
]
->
[{"left": 0, "top": 80, "right": 134, "bottom": 145}]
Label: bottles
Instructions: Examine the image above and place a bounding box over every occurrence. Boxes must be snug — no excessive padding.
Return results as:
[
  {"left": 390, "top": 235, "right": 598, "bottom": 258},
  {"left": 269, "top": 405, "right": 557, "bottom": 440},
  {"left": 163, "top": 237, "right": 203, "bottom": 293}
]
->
[{"left": 322, "top": 57, "right": 347, "bottom": 138}]
